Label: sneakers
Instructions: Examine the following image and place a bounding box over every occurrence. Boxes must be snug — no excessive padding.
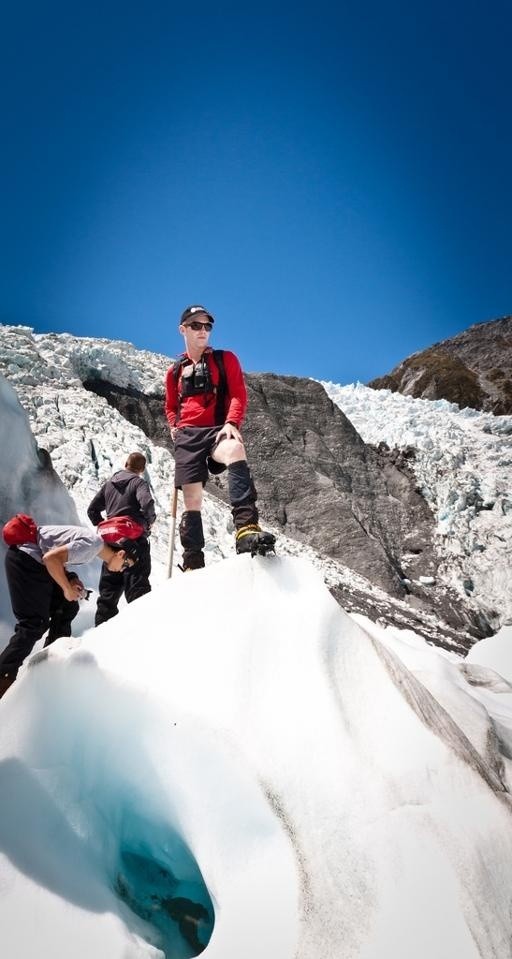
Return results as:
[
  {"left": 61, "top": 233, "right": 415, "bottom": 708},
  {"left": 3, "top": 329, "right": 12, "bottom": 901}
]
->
[{"left": 234, "top": 524, "right": 276, "bottom": 558}]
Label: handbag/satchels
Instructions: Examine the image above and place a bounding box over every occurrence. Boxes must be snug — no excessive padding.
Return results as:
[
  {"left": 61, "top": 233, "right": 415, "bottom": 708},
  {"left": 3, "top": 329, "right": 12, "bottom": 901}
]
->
[
  {"left": 96, "top": 516, "right": 145, "bottom": 543},
  {"left": 2, "top": 512, "right": 37, "bottom": 546}
]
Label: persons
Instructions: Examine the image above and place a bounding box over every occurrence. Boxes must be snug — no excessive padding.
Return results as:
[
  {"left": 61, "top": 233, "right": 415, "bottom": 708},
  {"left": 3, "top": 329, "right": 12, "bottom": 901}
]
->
[
  {"left": 1, "top": 524, "right": 141, "bottom": 698},
  {"left": 165, "top": 305, "right": 277, "bottom": 573},
  {"left": 87, "top": 452, "right": 157, "bottom": 627}
]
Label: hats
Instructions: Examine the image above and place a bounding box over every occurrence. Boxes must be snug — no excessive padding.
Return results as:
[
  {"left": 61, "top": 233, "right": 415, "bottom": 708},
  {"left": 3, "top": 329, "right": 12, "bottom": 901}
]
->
[
  {"left": 108, "top": 537, "right": 138, "bottom": 563},
  {"left": 179, "top": 305, "right": 214, "bottom": 325}
]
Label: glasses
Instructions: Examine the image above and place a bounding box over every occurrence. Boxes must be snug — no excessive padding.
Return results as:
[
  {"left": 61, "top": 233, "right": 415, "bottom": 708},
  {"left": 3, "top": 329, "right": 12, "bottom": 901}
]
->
[
  {"left": 122, "top": 555, "right": 129, "bottom": 570},
  {"left": 183, "top": 321, "right": 213, "bottom": 331}
]
